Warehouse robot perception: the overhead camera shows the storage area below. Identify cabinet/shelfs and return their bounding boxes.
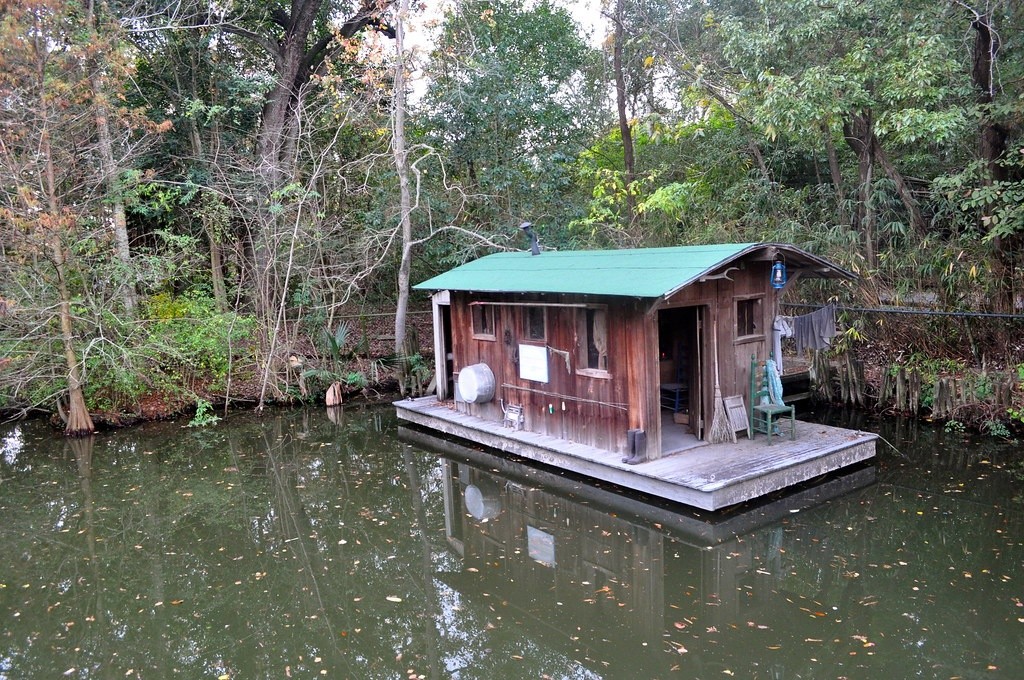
[659,381,687,414]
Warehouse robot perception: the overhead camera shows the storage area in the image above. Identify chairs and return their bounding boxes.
[747,353,794,446]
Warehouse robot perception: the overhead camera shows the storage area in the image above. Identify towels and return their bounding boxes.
[774,301,839,378]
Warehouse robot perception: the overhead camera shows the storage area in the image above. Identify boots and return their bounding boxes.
[622,429,646,464]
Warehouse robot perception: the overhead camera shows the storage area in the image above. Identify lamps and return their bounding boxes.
[770,249,787,289]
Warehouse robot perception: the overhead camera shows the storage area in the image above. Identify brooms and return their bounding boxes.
[706,320,738,445]
